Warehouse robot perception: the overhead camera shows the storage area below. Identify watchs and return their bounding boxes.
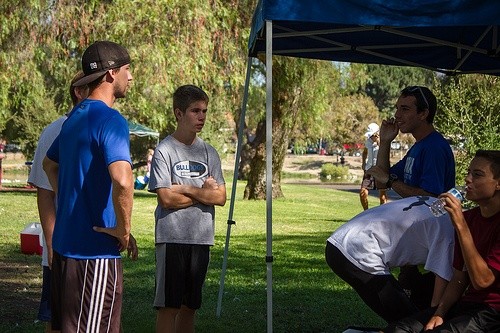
[386,173,398,189]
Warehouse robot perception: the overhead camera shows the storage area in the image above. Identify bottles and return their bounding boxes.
[429,186,470,217]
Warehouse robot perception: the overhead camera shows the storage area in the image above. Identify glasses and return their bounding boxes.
[401,86,429,109]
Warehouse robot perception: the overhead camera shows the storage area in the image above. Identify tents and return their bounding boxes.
[216,0,500,332]
[126,120,160,147]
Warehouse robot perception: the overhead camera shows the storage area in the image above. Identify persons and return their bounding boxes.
[423,148,500,333]
[149,84,227,333]
[362,86,456,333]
[389,140,411,158]
[28,70,94,332]
[42,40,134,332]
[325,194,457,333]
[146,148,155,179]
[360,122,387,213]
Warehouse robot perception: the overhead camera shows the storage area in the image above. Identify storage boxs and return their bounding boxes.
[20,222,44,255]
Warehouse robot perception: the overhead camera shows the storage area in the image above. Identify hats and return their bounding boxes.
[364,122,380,138]
[72,40,130,87]
